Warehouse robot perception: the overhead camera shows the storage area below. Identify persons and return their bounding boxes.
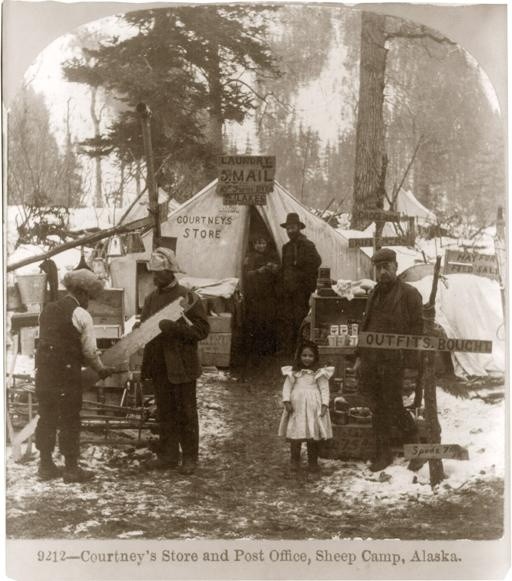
[278,340,336,472]
[33,268,115,484]
[270,212,322,361]
[230,232,282,378]
[133,247,210,475]
[345,248,429,471]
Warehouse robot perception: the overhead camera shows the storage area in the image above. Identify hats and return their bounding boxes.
[147,249,184,273]
[280,214,305,230]
[372,249,397,264]
[63,270,102,298]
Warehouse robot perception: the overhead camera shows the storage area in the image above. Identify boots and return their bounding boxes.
[404,431,429,471]
[368,431,395,472]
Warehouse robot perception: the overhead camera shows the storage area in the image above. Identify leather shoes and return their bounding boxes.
[180,460,196,475]
[145,459,180,470]
[38,464,63,482]
[62,470,97,484]
